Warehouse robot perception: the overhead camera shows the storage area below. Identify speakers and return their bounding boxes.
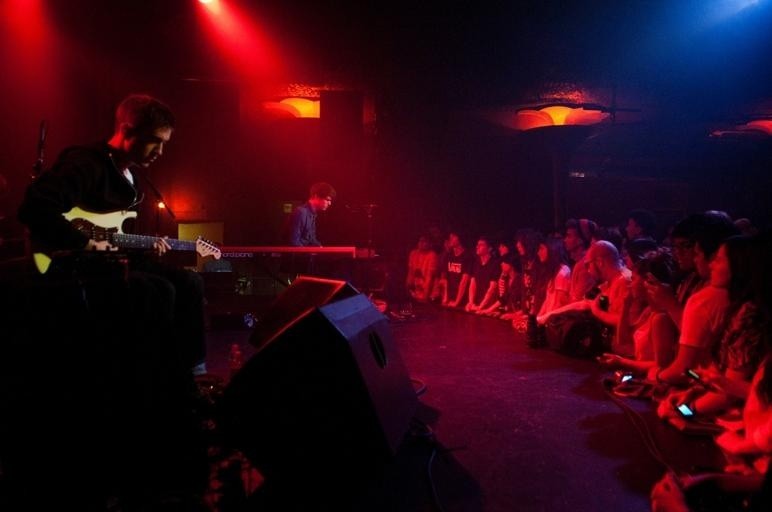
[554,318,600,357]
[219,293,421,511]
[248,272,360,354]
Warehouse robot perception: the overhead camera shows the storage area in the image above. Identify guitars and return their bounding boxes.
[61,206,221,276]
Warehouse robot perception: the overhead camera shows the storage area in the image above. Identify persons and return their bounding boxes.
[598,206,771,511]
[276,179,336,280]
[16,94,218,454]
[403,218,635,354]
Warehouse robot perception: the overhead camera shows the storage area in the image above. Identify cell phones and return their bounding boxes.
[687,368,702,382]
[620,373,634,382]
[673,404,695,418]
[638,267,653,285]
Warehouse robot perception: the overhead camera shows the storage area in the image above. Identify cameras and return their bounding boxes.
[585,287,608,308]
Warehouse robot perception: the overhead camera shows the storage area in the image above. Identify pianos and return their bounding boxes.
[215,241,360,263]
